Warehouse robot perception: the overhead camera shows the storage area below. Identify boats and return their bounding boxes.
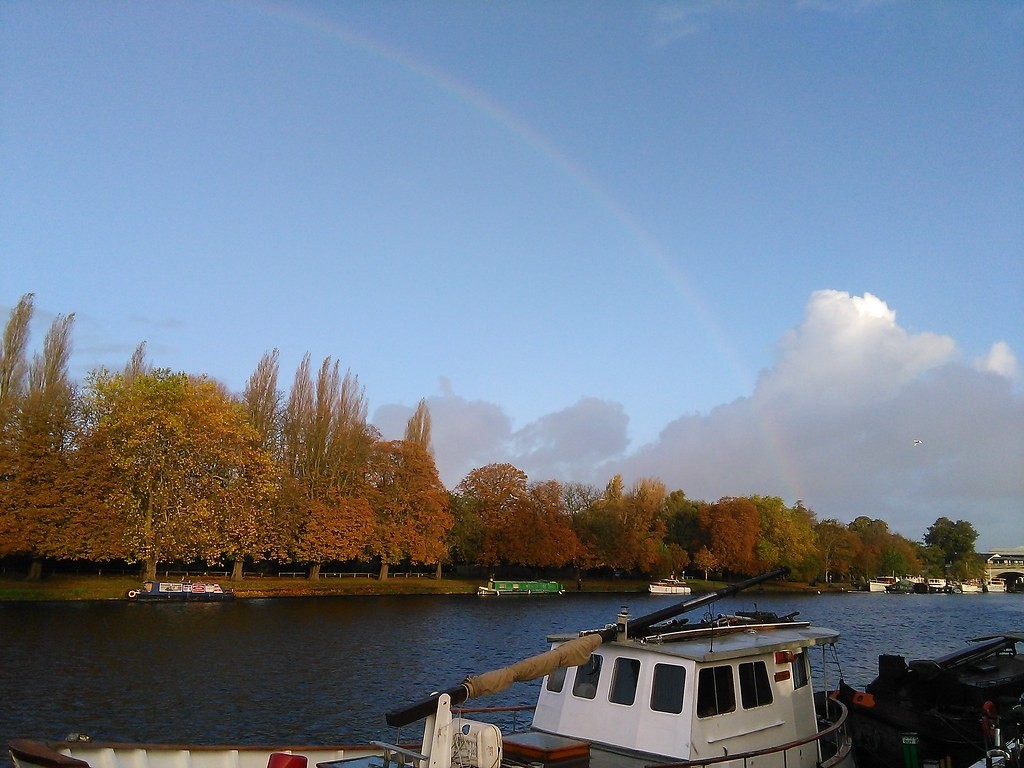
[648,571,691,594]
[478,574,565,595]
[869,565,1015,594]
[126,580,236,603]
[5,616,1023,768]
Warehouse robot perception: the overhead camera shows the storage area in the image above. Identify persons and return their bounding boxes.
[1010,692,1024,732]
[977,700,1007,748]
